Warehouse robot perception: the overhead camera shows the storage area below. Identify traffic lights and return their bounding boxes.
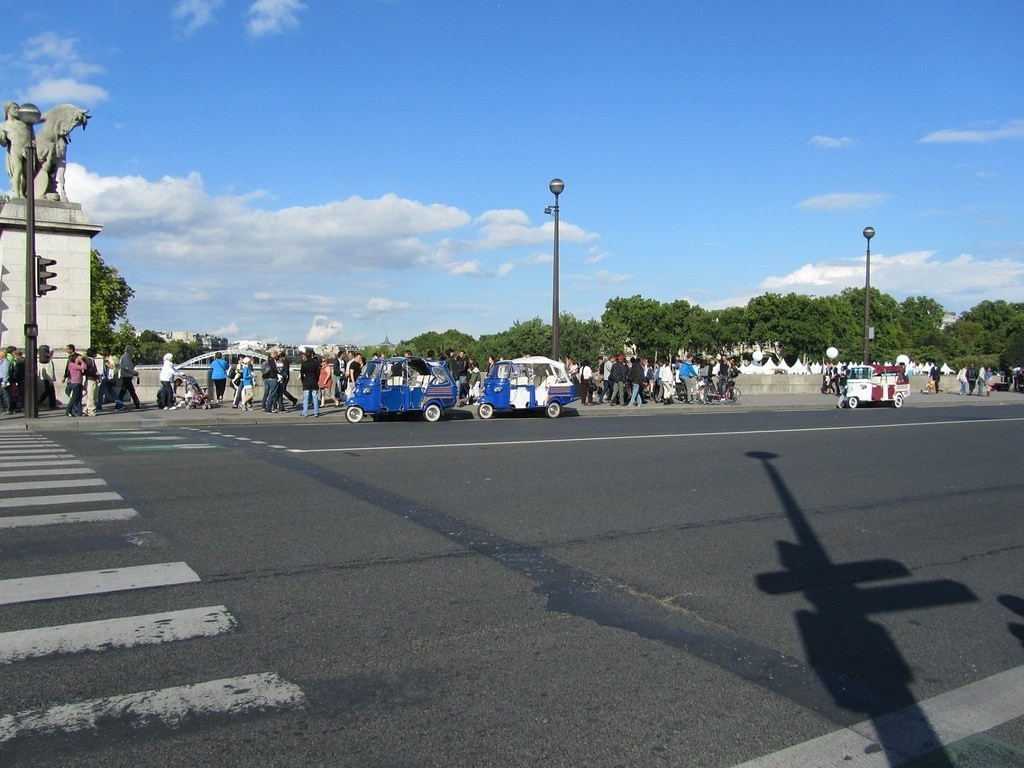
[38,257,56,296]
[39,345,51,363]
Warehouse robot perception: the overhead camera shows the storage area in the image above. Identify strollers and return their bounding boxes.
[182,375,212,410]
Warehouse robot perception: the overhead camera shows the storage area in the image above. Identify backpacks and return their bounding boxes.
[729,359,738,377]
[701,364,709,377]
[261,358,272,375]
[626,367,635,382]
[608,363,622,382]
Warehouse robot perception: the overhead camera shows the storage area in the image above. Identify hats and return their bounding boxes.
[243,357,251,363]
[445,349,454,356]
[618,355,624,360]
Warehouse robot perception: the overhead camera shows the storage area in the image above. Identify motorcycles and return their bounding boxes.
[846,365,911,409]
[346,355,459,423]
[477,356,574,419]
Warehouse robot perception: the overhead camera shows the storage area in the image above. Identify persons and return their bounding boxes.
[499,357,509,374]
[486,356,496,377]
[0,345,439,416]
[1005,366,1024,391]
[580,355,738,408]
[930,362,940,393]
[824,361,850,394]
[439,349,481,407]
[564,357,579,385]
[0,101,37,199]
[984,366,991,397]
[965,363,979,396]
[959,364,968,396]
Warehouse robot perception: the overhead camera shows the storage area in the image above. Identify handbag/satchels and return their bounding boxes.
[587,379,597,391]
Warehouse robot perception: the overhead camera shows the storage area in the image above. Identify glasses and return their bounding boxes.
[322,361,326,362]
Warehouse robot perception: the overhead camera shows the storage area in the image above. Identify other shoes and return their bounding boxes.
[629,403,633,407]
[164,407,168,409]
[664,399,669,405]
[293,399,298,407]
[599,400,604,403]
[319,405,325,408]
[96,406,103,411]
[65,412,73,416]
[335,400,340,407]
[217,400,219,403]
[219,396,222,401]
[279,409,289,414]
[2,410,13,415]
[959,393,961,394]
[232,404,238,408]
[667,398,674,404]
[689,398,695,403]
[135,405,147,410]
[473,401,480,406]
[986,391,991,397]
[50,406,59,409]
[262,407,265,411]
[610,400,613,405]
[239,404,246,410]
[117,405,126,411]
[169,406,177,410]
[463,400,469,405]
[638,404,646,407]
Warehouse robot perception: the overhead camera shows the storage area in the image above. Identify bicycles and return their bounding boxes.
[672,374,706,405]
[698,376,741,406]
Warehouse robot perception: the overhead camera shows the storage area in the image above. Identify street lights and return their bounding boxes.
[863,227,876,365]
[545,179,564,360]
[18,103,43,419]
